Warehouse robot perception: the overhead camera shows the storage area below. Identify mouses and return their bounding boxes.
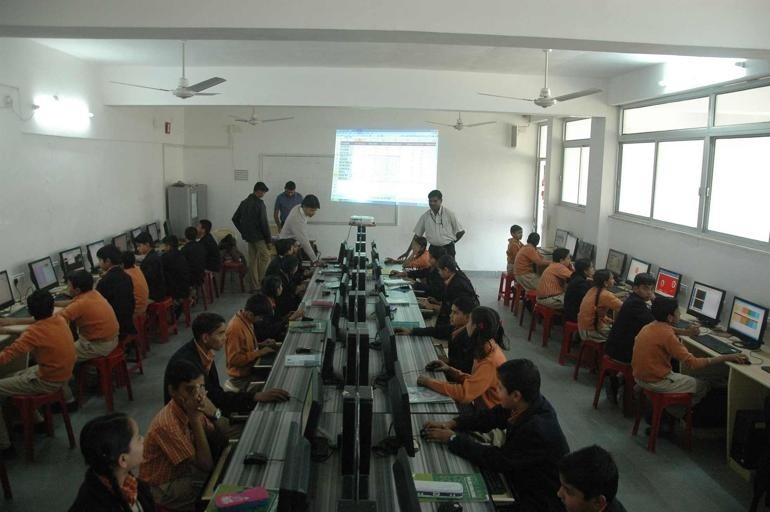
[295,344,312,353]
[243,451,268,465]
[322,290,330,296]
[399,284,410,289]
[742,358,751,365]
[437,501,463,512]
[301,315,315,322]
[420,426,430,438]
[425,363,440,370]
[319,263,329,268]
[315,278,325,283]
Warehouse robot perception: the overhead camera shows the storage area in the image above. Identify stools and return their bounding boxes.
[6,388,78,462]
[75,346,135,414]
[172,289,193,329]
[559,321,593,369]
[519,291,537,330]
[631,387,694,455]
[115,333,143,389]
[593,355,634,418]
[134,314,150,358]
[209,271,219,303]
[528,304,554,347]
[220,262,245,293]
[148,296,178,344]
[511,282,524,315]
[197,274,211,311]
[574,340,605,380]
[497,271,515,305]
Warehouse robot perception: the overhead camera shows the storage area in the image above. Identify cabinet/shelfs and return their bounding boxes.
[168,184,208,238]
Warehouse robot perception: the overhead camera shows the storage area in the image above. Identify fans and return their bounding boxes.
[423,111,497,131]
[233,107,294,125]
[110,39,225,99]
[478,47,603,108]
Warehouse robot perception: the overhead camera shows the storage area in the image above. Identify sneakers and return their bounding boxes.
[604,375,619,405]
[51,400,79,413]
[15,419,46,433]
[0,443,16,460]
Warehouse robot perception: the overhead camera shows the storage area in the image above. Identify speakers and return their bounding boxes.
[510,123,519,149]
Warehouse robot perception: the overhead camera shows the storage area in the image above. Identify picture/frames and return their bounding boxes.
[165,122,171,133]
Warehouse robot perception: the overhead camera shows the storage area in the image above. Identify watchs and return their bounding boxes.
[448,434,457,442]
[206,408,222,421]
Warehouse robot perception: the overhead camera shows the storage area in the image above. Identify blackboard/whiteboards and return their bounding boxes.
[258,154,398,227]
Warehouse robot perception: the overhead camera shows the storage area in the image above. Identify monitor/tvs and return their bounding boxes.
[727,296,769,349]
[146,222,160,243]
[654,267,682,299]
[337,240,348,268]
[299,366,329,457]
[371,257,382,281]
[339,272,349,300]
[130,226,142,241]
[370,246,379,263]
[86,239,105,274]
[370,240,376,249]
[163,219,172,237]
[564,234,578,258]
[59,246,85,277]
[374,274,389,297]
[320,320,337,377]
[686,280,727,326]
[112,233,128,253]
[605,248,628,276]
[574,239,595,262]
[391,445,422,512]
[276,421,319,512]
[28,255,59,291]
[329,290,344,329]
[375,291,391,328]
[378,315,398,376]
[340,257,349,279]
[0,270,15,311]
[625,257,651,289]
[383,360,420,458]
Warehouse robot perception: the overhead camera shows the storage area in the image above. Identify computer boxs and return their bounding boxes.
[348,290,356,321]
[690,386,728,431]
[730,408,766,471]
[357,290,366,322]
[355,242,366,252]
[358,385,374,475]
[352,269,358,290]
[353,253,366,269]
[345,321,357,385]
[358,270,366,291]
[358,322,370,386]
[341,386,357,475]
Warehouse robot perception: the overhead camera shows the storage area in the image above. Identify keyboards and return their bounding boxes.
[689,334,741,354]
[481,469,508,495]
[258,344,280,365]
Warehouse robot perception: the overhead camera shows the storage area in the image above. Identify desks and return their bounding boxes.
[252,301,460,413]
[222,411,496,512]
[673,307,770,482]
[301,258,418,304]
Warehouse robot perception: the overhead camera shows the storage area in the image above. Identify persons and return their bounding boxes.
[507,225,524,274]
[422,359,570,512]
[232,182,274,294]
[578,269,623,343]
[95,244,137,340]
[536,248,576,309]
[197,219,220,272]
[254,275,305,342]
[393,295,480,367]
[630,297,749,420]
[0,289,76,459]
[164,312,290,407]
[67,413,155,512]
[0,269,119,412]
[418,255,475,311]
[138,358,231,512]
[397,190,465,261]
[120,251,149,314]
[136,231,167,304]
[563,258,594,320]
[605,273,700,405]
[279,195,324,267]
[418,307,508,410]
[274,181,304,232]
[513,232,549,290]
[276,256,305,309]
[557,443,627,512]
[225,295,277,391]
[389,246,446,294]
[161,235,193,299]
[265,239,293,275]
[290,238,299,256]
[181,227,204,286]
[384,237,430,267]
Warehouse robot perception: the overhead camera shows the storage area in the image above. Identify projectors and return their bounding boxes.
[349,215,374,225]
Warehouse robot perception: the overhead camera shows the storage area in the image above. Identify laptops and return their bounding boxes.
[540,228,569,255]
